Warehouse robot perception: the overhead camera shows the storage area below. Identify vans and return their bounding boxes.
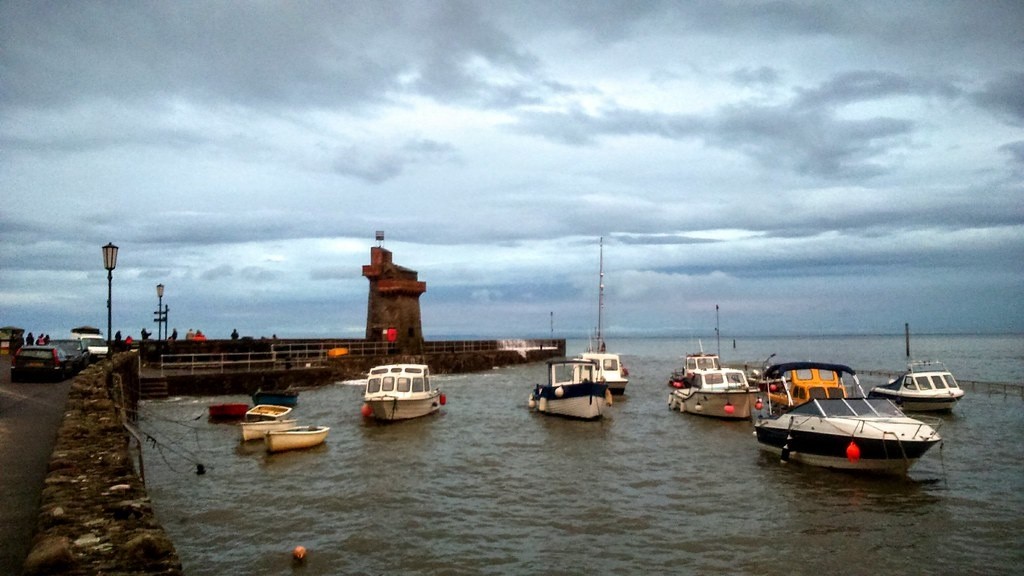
[69,326,111,358]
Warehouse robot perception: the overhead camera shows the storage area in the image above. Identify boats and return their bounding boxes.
[761,366,851,412]
[267,424,331,452]
[241,418,298,443]
[679,337,727,386]
[244,401,295,424]
[666,367,759,419]
[742,365,790,394]
[253,384,300,406]
[528,356,613,421]
[867,356,967,415]
[361,363,446,421]
[567,235,630,395]
[206,402,249,419]
[750,360,948,481]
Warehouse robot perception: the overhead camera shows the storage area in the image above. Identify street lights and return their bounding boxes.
[156,283,165,352]
[101,240,120,361]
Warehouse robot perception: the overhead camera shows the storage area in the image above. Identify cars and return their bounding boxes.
[9,344,77,382]
[48,338,90,370]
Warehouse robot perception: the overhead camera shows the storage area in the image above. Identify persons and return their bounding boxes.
[10,333,16,351]
[15,332,24,349]
[26,333,34,345]
[141,328,151,339]
[125,336,132,345]
[186,329,194,338]
[115,331,121,341]
[38,334,45,345]
[172,328,177,339]
[196,330,205,338]
[44,335,50,344]
[231,329,238,340]
